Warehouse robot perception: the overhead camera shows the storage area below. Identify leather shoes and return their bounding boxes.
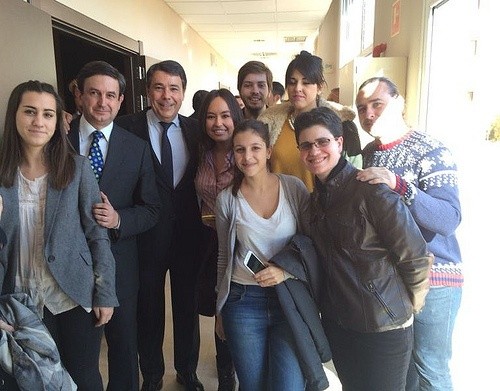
[141,379,163,391]
[176,374,204,391]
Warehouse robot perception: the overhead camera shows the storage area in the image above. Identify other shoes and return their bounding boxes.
[218,372,236,391]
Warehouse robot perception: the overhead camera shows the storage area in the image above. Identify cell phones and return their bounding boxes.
[243,249,268,274]
[201,214,216,222]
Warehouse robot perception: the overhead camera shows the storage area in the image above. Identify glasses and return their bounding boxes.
[297,137,338,151]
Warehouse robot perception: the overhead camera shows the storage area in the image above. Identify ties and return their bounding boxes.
[87,131,105,185]
[158,121,174,190]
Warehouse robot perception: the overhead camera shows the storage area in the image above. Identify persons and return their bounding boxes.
[0,49,464,391]
